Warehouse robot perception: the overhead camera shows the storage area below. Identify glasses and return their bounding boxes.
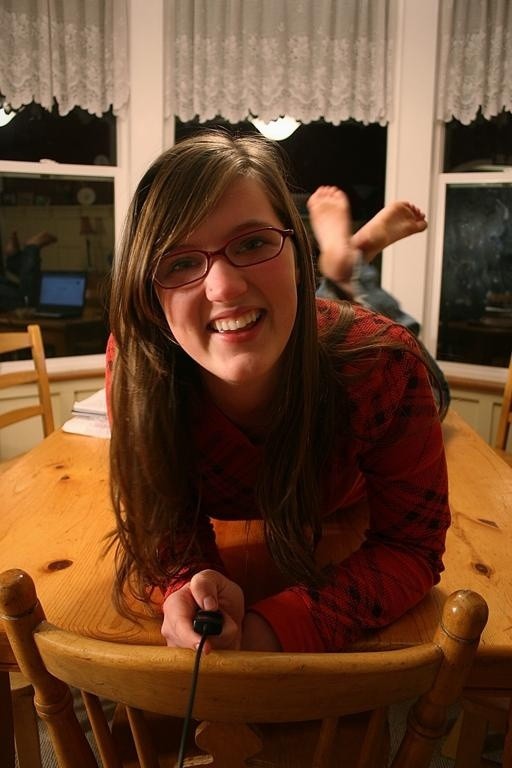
[148,226,295,289]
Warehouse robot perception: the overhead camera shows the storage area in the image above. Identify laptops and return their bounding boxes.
[32,270,88,318]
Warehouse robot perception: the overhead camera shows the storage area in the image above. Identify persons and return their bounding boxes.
[99,127,454,656]
[1,228,60,315]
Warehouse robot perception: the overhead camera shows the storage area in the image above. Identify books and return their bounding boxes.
[61,386,113,437]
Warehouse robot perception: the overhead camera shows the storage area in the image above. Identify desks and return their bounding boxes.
[1,363,512,738]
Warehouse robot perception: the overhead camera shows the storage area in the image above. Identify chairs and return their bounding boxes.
[492,361,512,463]
[1,563,492,767]
[2,322,55,461]
